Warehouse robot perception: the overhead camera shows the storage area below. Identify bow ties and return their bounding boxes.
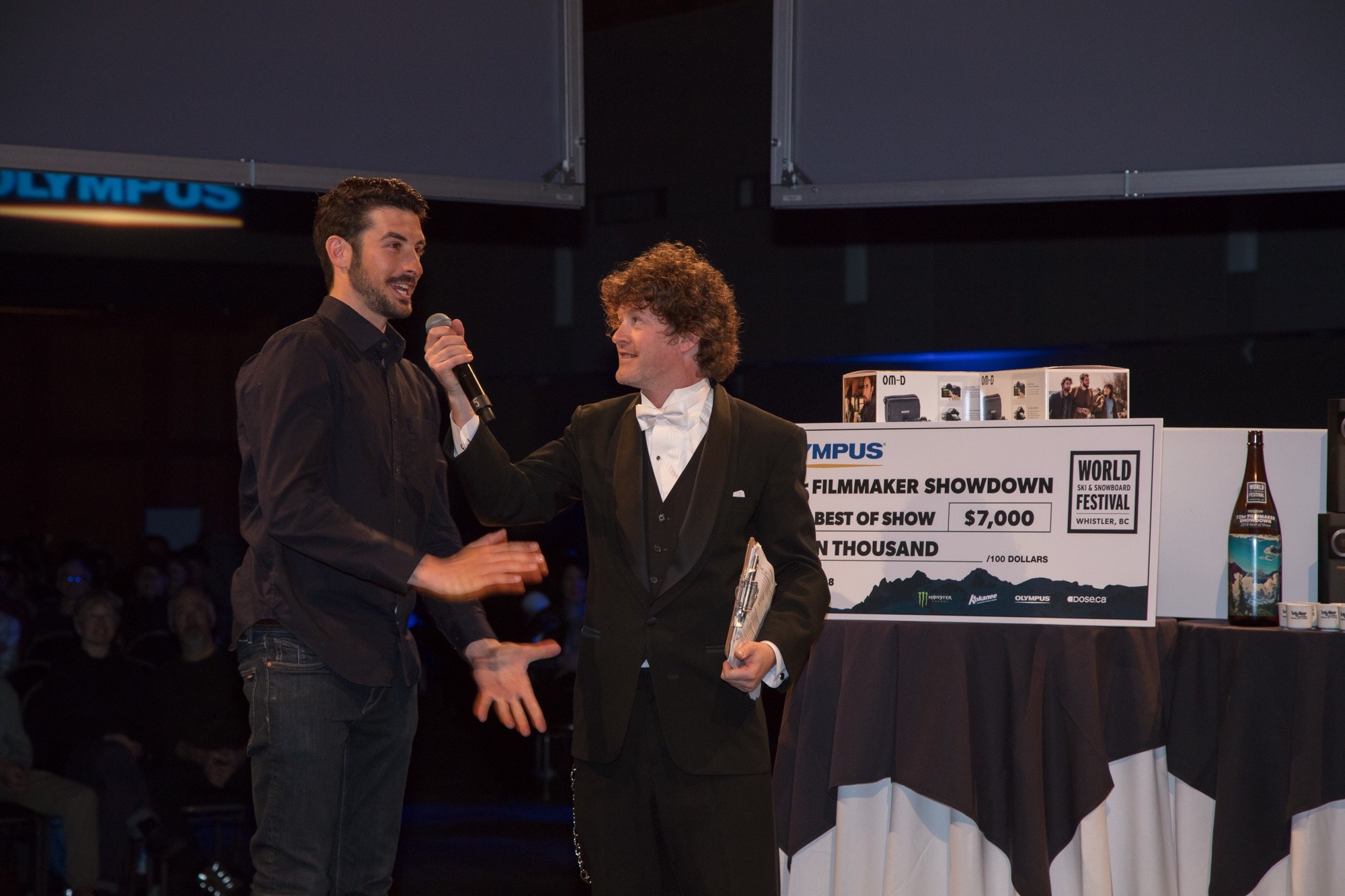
[636,401,688,431]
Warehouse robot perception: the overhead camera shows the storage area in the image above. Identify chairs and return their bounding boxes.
[0,803,247,896]
[530,663,574,801]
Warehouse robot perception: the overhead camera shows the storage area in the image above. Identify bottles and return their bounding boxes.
[1228,431,1282,627]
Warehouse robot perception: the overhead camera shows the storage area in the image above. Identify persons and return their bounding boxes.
[845,375,876,423]
[231,175,562,896]
[0,529,221,896]
[423,241,831,896]
[1049,374,1118,419]
[40,590,182,896]
[151,585,256,896]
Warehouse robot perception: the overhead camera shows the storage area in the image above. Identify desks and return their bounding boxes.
[770,614,1345,896]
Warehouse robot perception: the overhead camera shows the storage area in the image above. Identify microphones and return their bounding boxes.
[425,313,497,426]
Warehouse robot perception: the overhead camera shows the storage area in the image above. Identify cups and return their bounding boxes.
[1277,603,1293,627]
[1285,604,1313,628]
[1298,602,1320,627]
[1316,604,1339,631]
[1330,603,1345,605]
[1337,605,1345,633]
